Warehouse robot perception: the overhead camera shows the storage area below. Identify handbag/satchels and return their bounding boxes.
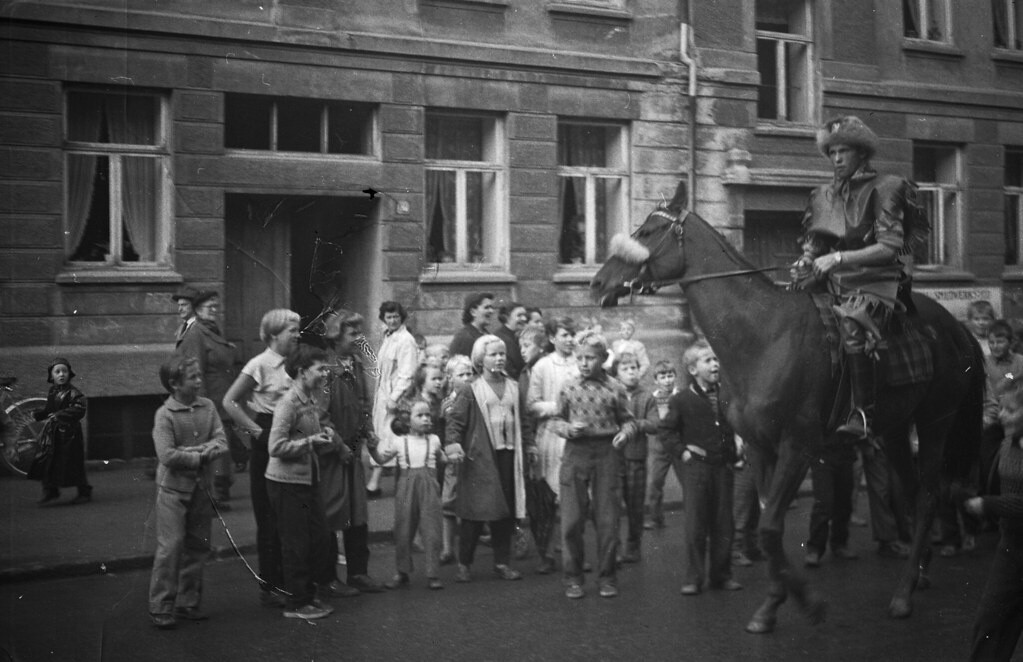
[26,420,48,479]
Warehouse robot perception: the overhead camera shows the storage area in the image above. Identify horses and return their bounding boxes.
[590,179,986,635]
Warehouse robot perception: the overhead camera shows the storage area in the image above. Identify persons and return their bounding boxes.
[32,357,97,509]
[149,353,229,630]
[546,330,637,598]
[365,300,420,500]
[785,115,908,442]
[174,287,1023,662]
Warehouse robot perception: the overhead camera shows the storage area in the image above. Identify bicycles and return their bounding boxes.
[0,376,49,477]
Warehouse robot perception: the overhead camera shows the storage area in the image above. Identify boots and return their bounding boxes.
[837,353,876,443]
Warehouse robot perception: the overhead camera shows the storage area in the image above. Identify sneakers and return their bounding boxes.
[283,596,335,619]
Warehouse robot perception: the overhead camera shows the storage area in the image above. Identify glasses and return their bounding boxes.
[199,304,219,308]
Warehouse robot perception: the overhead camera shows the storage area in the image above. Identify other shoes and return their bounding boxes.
[600,583,616,597]
[565,584,585,598]
[708,578,742,590]
[39,494,65,506]
[679,583,702,594]
[260,584,288,608]
[346,573,378,592]
[615,514,962,565]
[147,609,176,626]
[175,605,209,620]
[74,494,92,504]
[384,556,593,590]
[321,577,360,596]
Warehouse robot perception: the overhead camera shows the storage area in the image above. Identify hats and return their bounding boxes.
[172,286,199,301]
[817,115,880,164]
[192,291,213,309]
[47,358,76,383]
